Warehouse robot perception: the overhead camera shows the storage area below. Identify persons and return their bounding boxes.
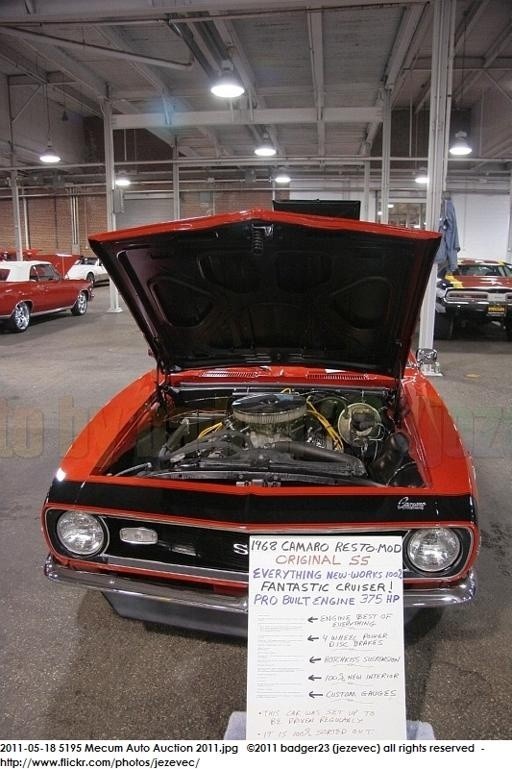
[23,252,28,261]
[1,251,11,261]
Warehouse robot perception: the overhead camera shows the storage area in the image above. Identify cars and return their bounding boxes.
[435,259,512,341]
[0,248,111,334]
[39,208,483,642]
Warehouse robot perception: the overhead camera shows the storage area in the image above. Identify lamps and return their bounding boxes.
[38,81,61,163]
[449,9,473,157]
[254,122,276,157]
[210,47,246,100]
